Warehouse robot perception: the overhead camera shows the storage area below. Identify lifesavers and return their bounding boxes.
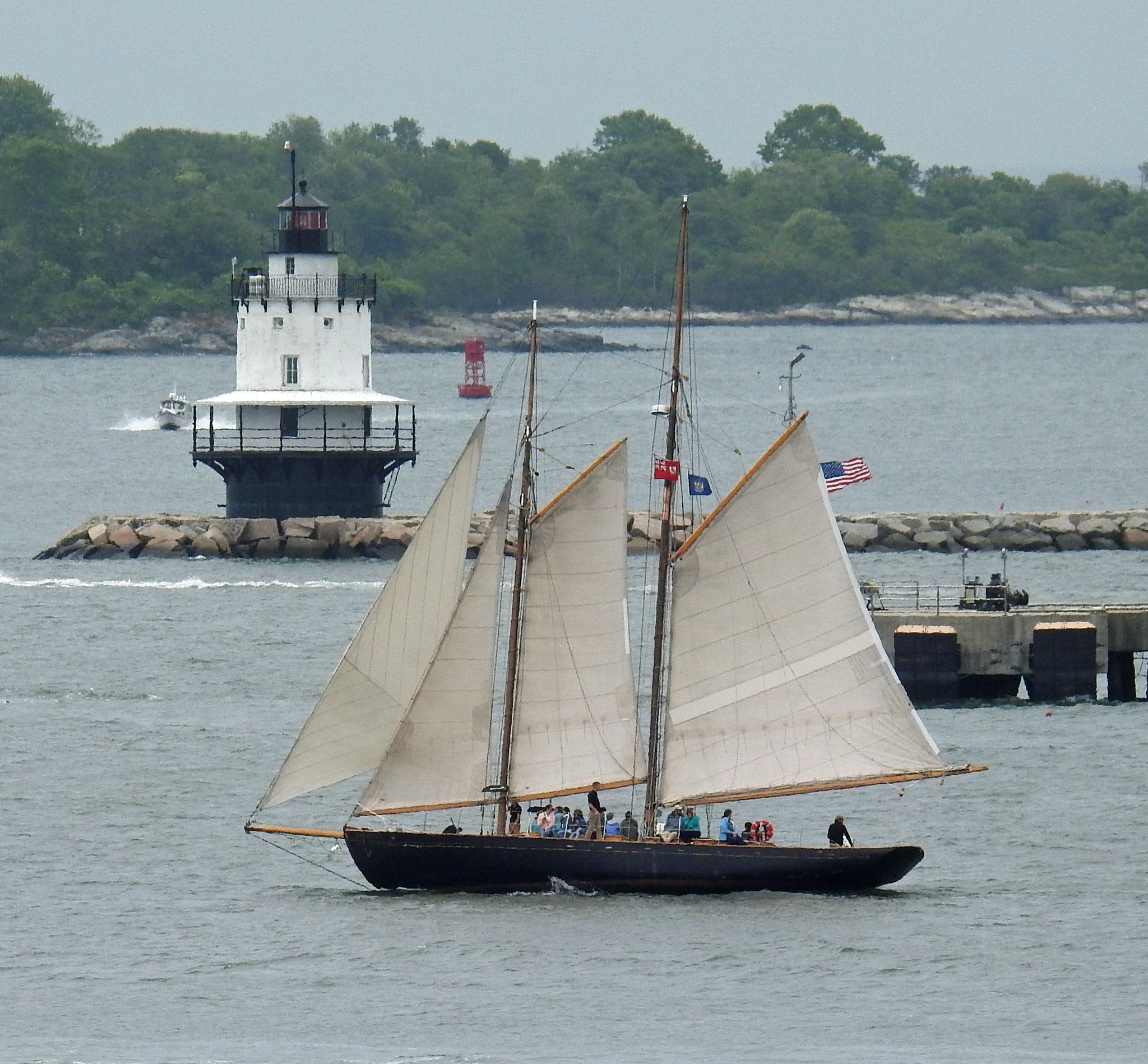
[750,819,773,843]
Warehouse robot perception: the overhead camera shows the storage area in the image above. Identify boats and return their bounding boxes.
[457,338,493,399]
[157,389,191,431]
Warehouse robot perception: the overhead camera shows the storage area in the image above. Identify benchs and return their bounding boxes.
[513,833,542,838]
[605,834,717,846]
[744,840,776,847]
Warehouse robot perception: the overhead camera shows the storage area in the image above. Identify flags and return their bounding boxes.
[688,474,712,496]
[654,458,680,481]
[820,457,872,492]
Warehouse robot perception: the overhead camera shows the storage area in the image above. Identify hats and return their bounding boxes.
[673,805,683,811]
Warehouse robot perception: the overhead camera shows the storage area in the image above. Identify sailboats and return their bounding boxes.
[244,192,991,894]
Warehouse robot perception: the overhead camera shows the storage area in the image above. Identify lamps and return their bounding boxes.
[232,256,237,279]
[651,405,669,415]
[284,141,293,153]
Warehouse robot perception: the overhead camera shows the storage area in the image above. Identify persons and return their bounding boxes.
[531,804,587,840]
[618,811,639,840]
[506,801,522,833]
[719,809,744,846]
[989,573,1004,586]
[827,815,853,848]
[658,804,686,842]
[583,781,603,841]
[680,807,701,843]
[606,812,620,836]
[741,822,753,839]
